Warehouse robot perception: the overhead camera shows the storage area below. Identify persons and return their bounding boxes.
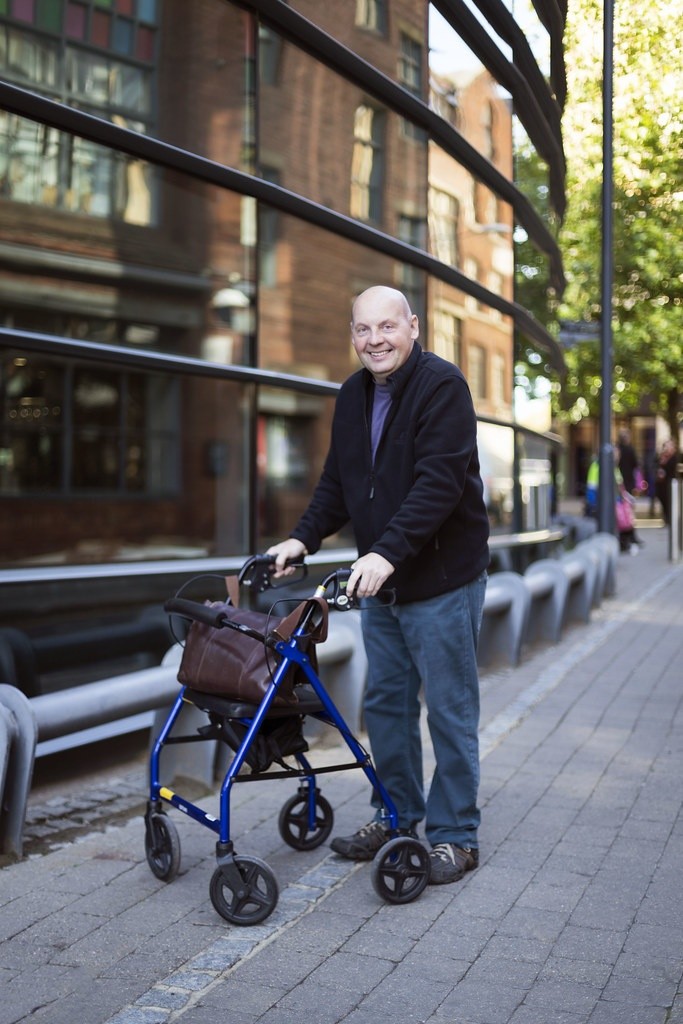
[267,284,491,886]
[584,427,683,557]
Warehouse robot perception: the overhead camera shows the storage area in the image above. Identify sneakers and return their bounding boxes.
[330,809,418,861]
[427,842,479,884]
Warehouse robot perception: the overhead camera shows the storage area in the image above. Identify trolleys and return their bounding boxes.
[141,553,434,928]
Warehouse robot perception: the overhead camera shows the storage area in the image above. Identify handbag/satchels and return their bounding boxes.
[177,575,329,708]
[615,501,635,533]
